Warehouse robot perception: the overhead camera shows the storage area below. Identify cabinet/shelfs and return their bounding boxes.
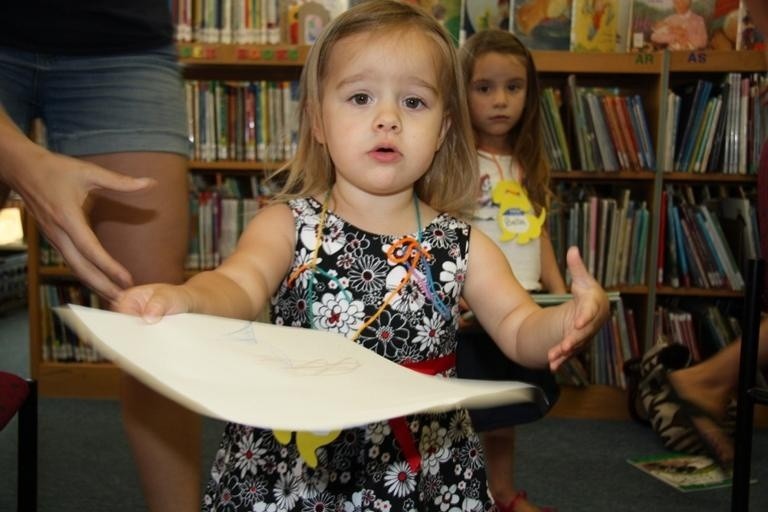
[27,1,766,421]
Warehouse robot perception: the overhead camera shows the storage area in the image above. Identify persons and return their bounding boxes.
[112,1,613,509]
[0,1,203,512]
[625,312,768,461]
[0,99,157,305]
[459,29,569,510]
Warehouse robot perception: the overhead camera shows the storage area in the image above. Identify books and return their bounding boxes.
[538,87,654,389]
[656,72,767,366]
[300,0,767,53]
[175,0,302,270]
[40,237,111,365]
